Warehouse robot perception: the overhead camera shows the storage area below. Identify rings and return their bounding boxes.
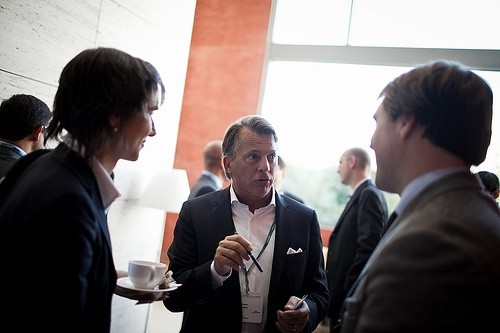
[294,324,297,332]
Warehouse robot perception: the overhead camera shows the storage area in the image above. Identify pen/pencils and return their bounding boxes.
[234,230,263,273]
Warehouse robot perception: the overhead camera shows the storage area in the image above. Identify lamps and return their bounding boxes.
[139,168,190,333]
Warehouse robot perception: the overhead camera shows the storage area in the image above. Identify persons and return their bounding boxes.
[186,140,225,202]
[325,147,388,333]
[275,156,305,205]
[0,47,166,333]
[0,94,52,193]
[166,113,330,333]
[341,60,500,333]
[477,171,500,201]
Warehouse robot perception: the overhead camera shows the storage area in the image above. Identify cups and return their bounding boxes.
[128,260,167,290]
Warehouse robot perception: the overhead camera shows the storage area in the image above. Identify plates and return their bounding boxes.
[117,276,178,293]
